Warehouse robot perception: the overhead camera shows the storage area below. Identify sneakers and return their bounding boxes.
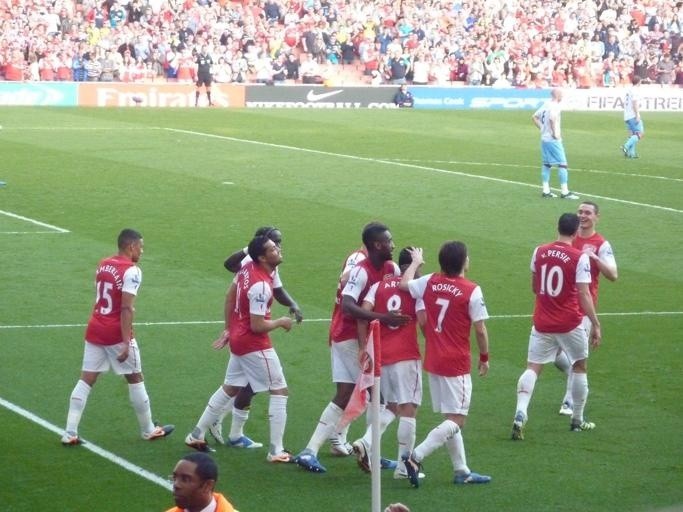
[559,401,574,416]
[562,192,580,202]
[184,430,426,489]
[512,415,524,441]
[142,424,176,441]
[571,422,597,432]
[543,192,558,199]
[454,469,493,485]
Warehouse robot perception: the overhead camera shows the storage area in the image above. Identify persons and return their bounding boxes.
[531,87,583,201]
[554,202,617,416]
[62,228,176,448]
[191,42,217,108]
[617,81,646,161]
[392,81,416,108]
[162,450,240,512]
[292,220,491,489]
[185,226,302,463]
[512,213,602,441]
[1,0,682,84]
